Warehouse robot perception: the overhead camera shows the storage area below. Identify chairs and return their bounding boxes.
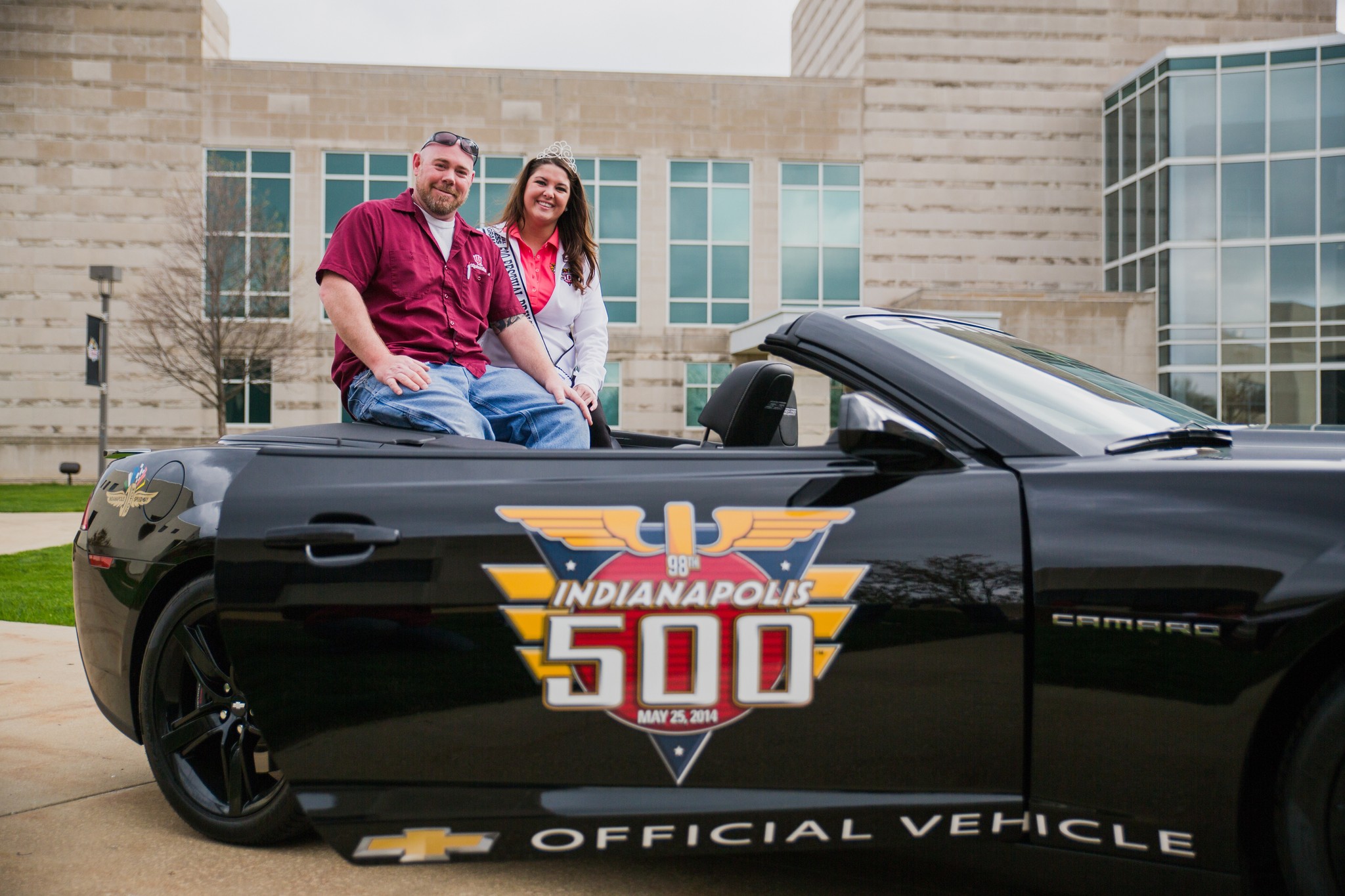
[672,360,799,453]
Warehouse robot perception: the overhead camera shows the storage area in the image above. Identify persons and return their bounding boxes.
[474,151,614,450]
[315,132,593,450]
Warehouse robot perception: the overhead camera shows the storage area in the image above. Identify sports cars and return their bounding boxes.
[68,305,1345,896]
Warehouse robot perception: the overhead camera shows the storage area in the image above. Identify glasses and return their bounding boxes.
[422,132,479,167]
